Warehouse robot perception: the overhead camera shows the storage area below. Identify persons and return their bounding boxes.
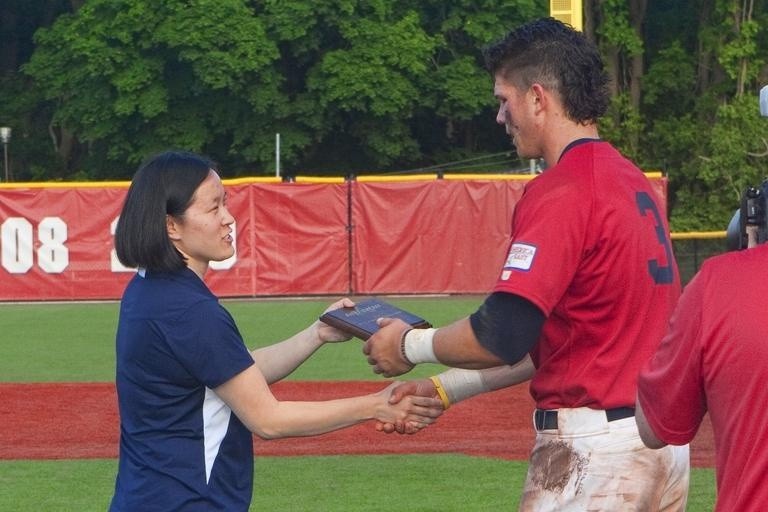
[106,151,448,512]
[363,18,691,512]
[633,239,768,512]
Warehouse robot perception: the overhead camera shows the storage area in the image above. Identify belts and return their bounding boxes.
[529,402,637,433]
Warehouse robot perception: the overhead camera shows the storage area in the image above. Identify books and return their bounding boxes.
[318,297,432,343]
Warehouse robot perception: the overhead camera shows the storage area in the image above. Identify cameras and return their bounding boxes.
[741,186,766,227]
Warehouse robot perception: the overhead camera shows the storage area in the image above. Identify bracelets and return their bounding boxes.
[430,376,450,409]
[401,325,439,369]
